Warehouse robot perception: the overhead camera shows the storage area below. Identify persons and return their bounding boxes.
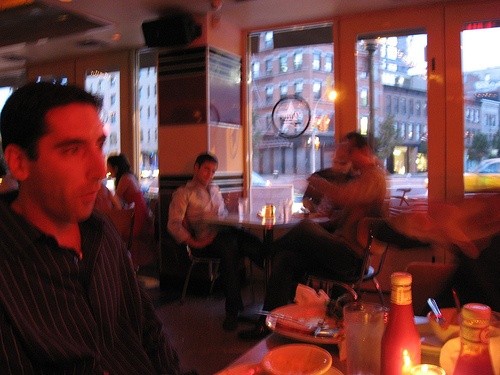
[237,131,391,343]
[0,80,181,375]
[167,154,264,331]
[106,154,154,272]
[93,181,131,237]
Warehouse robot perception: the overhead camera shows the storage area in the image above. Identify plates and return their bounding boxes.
[438,337,500,375]
[417,334,442,356]
[214,362,345,375]
[266,301,343,344]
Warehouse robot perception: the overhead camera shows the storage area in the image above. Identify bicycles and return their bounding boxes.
[391,187,411,208]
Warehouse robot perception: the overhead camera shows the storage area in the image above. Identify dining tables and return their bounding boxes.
[212,319,447,375]
[203,214,331,316]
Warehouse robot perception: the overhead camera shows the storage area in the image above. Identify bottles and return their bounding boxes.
[381,271,421,375]
[451,303,496,375]
[263,201,274,219]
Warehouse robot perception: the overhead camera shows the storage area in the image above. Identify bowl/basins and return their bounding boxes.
[264,344,332,375]
[427,307,500,344]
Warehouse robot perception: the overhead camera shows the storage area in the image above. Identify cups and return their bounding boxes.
[409,363,448,375]
[279,201,292,222]
[238,197,250,218]
[343,302,389,375]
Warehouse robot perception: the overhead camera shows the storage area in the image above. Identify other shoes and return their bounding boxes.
[223,317,238,331]
[237,321,268,342]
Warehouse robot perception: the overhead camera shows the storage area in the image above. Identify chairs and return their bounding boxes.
[179,214,222,305]
[302,216,389,308]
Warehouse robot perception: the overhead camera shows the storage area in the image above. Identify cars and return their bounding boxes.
[140,171,304,203]
[424,158,500,193]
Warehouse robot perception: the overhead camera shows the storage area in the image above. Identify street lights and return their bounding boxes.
[311,91,337,174]
[364,39,379,150]
